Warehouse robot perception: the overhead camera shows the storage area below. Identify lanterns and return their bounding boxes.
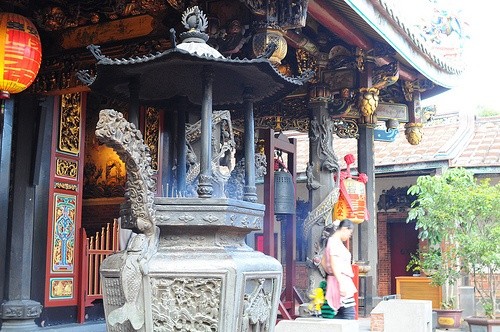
[331,178,369,225]
[0,11,44,99]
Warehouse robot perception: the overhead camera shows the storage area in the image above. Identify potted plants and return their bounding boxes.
[354,259,370,277]
[432,297,465,330]
[404,168,500,332]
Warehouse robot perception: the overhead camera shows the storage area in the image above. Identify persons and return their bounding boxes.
[314,220,360,319]
